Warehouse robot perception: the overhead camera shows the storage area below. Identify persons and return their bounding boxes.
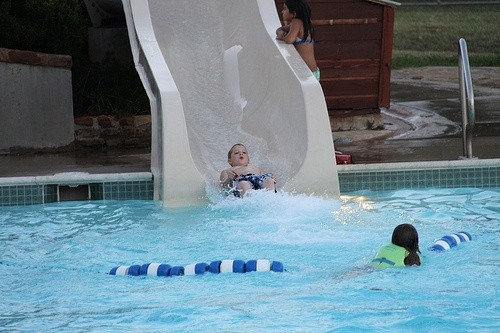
[276,0,320,82]
[219,143,277,200]
[339,223,421,280]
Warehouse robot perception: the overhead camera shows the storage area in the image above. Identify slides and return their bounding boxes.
[122,0,342,209]
